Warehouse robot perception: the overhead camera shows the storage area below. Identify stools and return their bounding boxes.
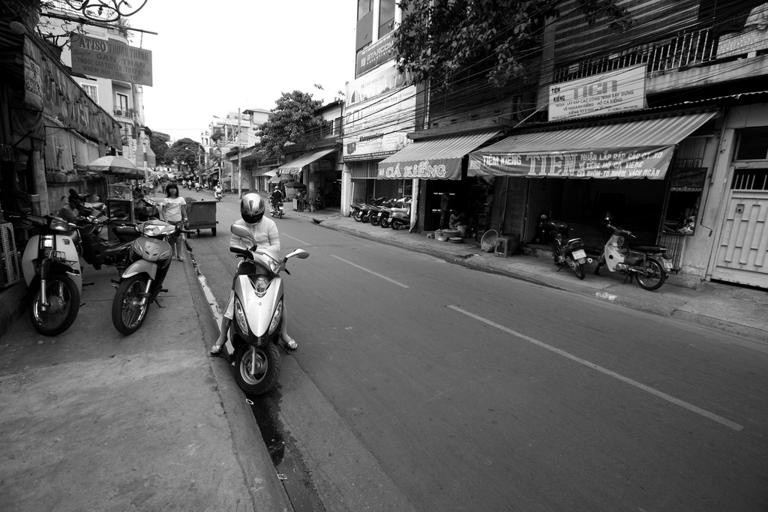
[495,236,513,257]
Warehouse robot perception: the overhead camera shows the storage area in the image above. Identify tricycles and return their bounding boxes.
[182,199,218,238]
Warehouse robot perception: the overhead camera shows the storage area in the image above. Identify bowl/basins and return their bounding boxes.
[479,228,498,252]
[450,237,462,243]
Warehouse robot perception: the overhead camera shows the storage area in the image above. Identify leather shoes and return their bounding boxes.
[281,334,298,350]
[211,337,228,354]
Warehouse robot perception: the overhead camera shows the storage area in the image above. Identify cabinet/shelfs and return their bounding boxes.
[109,183,132,200]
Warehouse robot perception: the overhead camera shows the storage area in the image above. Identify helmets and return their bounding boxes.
[240,193,265,224]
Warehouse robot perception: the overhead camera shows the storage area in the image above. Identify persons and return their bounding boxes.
[270,186,284,213]
[159,184,189,262]
[58,195,101,271]
[213,182,224,197]
[438,191,451,229]
[210,193,298,354]
[449,211,464,229]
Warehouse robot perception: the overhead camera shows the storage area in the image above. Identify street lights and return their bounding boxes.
[213,114,237,145]
[186,148,197,161]
[243,109,275,116]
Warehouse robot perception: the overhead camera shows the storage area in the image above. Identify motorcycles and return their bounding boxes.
[541,214,591,281]
[60,190,145,271]
[5,209,89,337]
[181,182,224,200]
[349,194,411,231]
[112,207,200,335]
[268,197,284,220]
[593,214,676,291]
[224,225,310,394]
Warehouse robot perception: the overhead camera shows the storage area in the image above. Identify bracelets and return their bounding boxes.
[184,218,187,220]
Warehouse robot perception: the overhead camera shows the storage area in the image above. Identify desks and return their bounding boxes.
[106,198,134,237]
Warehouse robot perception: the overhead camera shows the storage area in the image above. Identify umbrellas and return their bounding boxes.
[88,155,145,199]
